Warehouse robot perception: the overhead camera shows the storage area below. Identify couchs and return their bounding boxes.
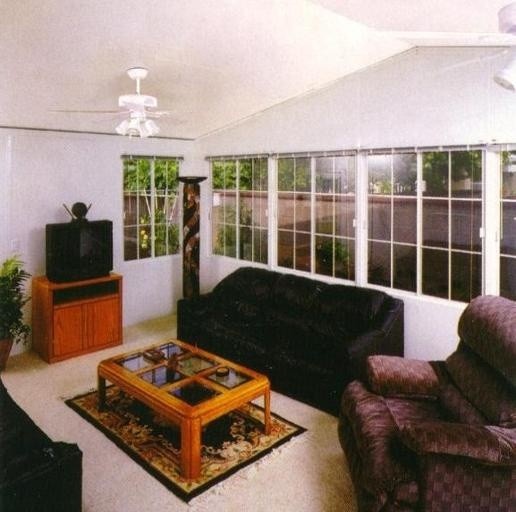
[0,375,83,512]
[176,266,405,418]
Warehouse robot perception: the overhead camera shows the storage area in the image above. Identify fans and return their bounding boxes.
[442,2,516,72]
[45,67,202,119]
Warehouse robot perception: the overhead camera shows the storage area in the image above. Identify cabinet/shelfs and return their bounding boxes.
[30,274,123,364]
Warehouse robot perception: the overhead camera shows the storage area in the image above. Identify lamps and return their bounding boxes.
[114,119,162,139]
[492,54,516,92]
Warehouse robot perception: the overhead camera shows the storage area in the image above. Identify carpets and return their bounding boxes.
[64,366,309,504]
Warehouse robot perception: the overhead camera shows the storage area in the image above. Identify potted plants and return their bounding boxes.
[0,253,33,372]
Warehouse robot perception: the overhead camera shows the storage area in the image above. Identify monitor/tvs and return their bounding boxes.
[46,221,112,283]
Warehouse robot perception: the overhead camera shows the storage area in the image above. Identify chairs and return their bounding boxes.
[336,293,516,512]
